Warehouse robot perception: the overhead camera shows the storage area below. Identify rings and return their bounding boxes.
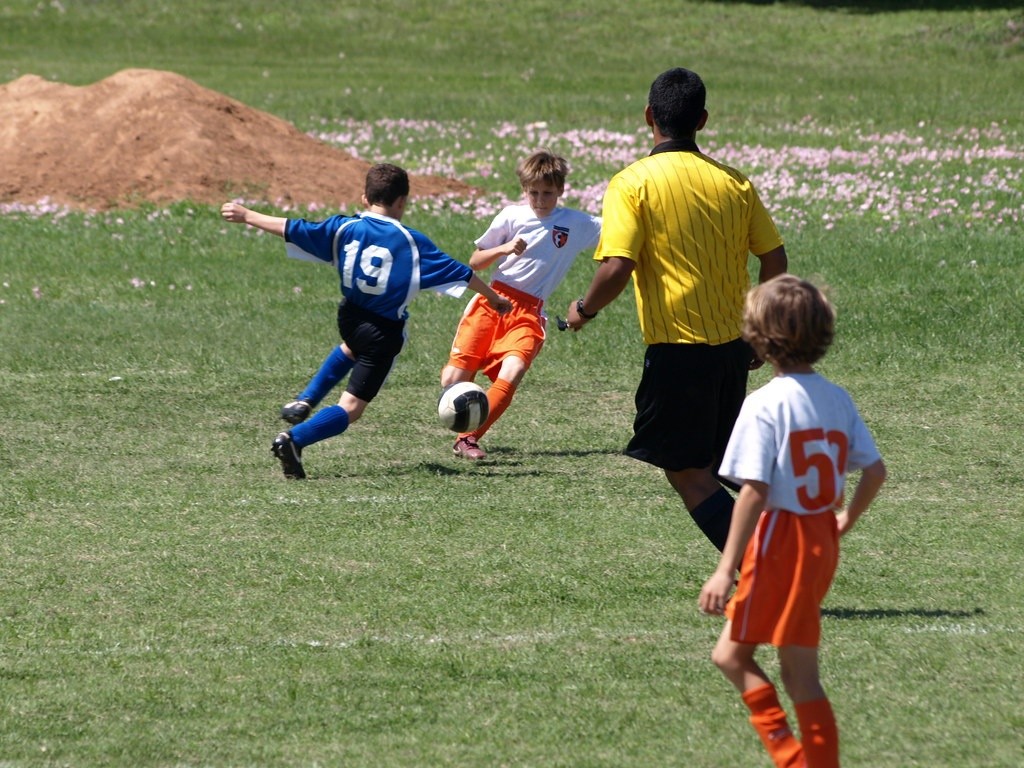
[567,324,570,328]
[566,319,568,323]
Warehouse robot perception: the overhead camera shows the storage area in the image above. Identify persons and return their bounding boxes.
[698,273,888,768]
[441,153,602,460]
[568,68,787,574]
[221,163,514,480]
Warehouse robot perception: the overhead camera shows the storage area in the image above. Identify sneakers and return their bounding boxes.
[452,435,487,459]
[281,401,312,425]
[271,431,306,480]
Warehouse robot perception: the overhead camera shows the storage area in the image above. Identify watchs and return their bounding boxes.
[576,299,597,319]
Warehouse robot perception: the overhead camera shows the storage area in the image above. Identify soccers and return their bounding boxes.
[436,381,490,434]
